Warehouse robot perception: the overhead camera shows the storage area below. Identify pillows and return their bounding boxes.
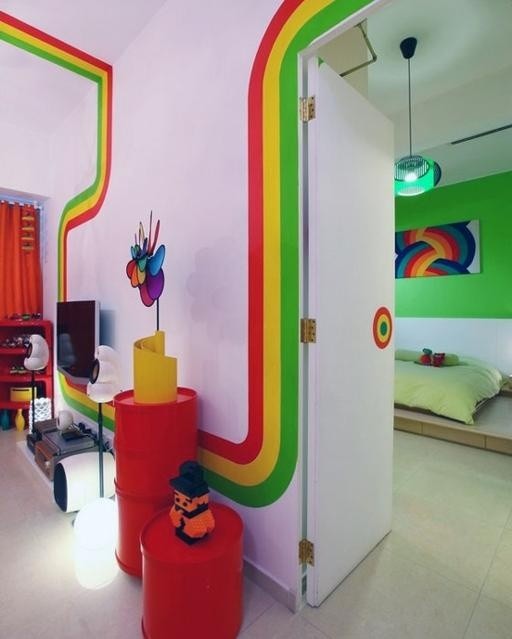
[414,352,459,366]
[395,349,422,360]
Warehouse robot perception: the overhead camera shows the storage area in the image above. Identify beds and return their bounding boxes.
[395,358,511,426]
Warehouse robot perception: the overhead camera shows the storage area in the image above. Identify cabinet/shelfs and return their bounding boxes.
[0,319,53,428]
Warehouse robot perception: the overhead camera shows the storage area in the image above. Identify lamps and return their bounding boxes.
[395,37,442,197]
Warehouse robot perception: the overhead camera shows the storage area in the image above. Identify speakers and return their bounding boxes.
[53,451,116,514]
[54,410,74,432]
[85,344,122,404]
[24,334,51,372]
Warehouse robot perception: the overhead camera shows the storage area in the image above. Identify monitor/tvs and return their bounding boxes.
[54,299,101,387]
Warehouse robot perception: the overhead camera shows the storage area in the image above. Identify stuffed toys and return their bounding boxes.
[420,347,433,364]
[433,351,447,368]
